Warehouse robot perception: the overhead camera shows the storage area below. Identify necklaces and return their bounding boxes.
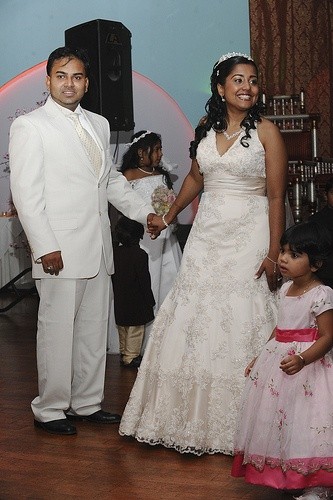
[138,168,155,174]
[289,280,315,296]
[222,129,243,139]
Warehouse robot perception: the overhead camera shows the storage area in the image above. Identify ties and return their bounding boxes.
[68,114,103,180]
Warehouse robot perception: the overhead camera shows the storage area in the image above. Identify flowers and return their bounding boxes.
[151,185,176,217]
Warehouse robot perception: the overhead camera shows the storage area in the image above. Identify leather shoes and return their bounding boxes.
[33,417,77,436]
[63,407,120,426]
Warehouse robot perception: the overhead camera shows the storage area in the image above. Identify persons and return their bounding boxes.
[118,52,295,456]
[8,47,157,435]
[231,220,333,500]
[107,131,183,357]
[110,216,157,368]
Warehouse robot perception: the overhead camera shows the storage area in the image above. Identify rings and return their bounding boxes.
[48,266,53,270]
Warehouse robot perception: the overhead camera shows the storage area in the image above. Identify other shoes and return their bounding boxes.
[123,355,143,368]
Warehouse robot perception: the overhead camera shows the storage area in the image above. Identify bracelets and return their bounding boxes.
[267,257,278,273]
[162,215,168,226]
[299,355,306,365]
